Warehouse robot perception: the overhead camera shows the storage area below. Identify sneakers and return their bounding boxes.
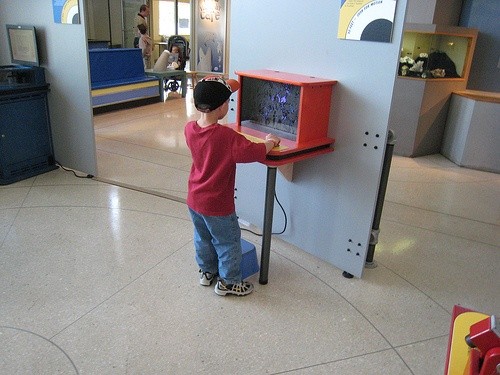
[199,269,217,286]
[215,279,254,296]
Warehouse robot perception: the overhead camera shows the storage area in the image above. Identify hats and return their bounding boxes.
[193,78,240,113]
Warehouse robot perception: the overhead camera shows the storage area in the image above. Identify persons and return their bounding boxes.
[184,74,280,297]
[134,5,150,48]
[172,45,182,70]
[138,24,152,69]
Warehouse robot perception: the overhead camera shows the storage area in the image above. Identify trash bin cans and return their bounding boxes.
[0,65,59,186]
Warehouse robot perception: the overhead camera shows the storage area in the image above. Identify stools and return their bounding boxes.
[186,71,199,90]
[238,237,260,281]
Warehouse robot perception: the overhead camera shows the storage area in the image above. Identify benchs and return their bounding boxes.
[88,47,160,111]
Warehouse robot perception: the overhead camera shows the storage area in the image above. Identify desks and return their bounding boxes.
[439,89,500,174]
[219,123,336,285]
[0,65,59,186]
[146,70,186,102]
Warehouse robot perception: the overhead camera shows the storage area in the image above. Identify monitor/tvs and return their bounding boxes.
[5,23,40,68]
